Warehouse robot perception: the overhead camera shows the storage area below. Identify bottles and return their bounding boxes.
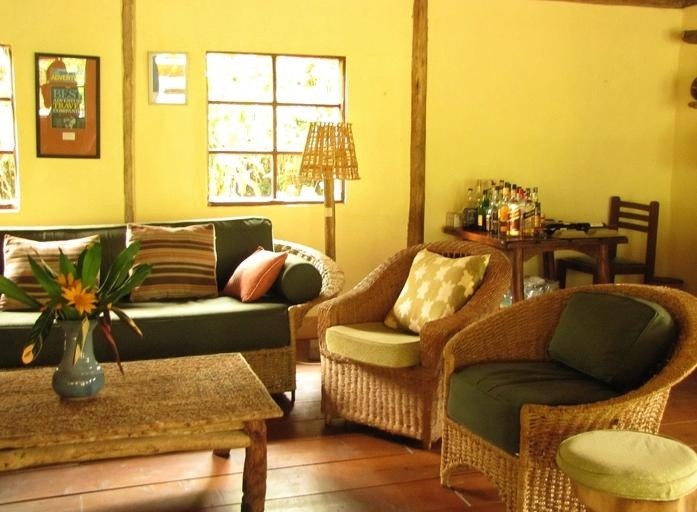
[462,178,542,238]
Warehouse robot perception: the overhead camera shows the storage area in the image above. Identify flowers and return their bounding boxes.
[0,242,153,380]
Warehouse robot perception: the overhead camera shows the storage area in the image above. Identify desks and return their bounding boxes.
[443,226,630,304]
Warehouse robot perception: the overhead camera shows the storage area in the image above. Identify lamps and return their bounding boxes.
[298,120,360,264]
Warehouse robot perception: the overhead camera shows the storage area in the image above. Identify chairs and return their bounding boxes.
[555,197,660,289]
[440,284,697,512]
[317,240,513,451]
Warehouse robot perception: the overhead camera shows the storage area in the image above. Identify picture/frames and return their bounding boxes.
[146,50,188,106]
[34,51,100,159]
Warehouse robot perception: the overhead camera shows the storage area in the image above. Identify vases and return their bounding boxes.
[52,318,106,401]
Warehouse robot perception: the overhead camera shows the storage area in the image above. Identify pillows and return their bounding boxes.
[0,234,101,310]
[125,222,218,302]
[546,291,678,392]
[264,253,323,303]
[383,247,491,335]
[224,246,288,303]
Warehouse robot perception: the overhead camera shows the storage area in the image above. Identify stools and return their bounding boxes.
[556,430,697,512]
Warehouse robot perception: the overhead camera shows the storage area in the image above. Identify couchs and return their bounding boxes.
[0,216,345,402]
